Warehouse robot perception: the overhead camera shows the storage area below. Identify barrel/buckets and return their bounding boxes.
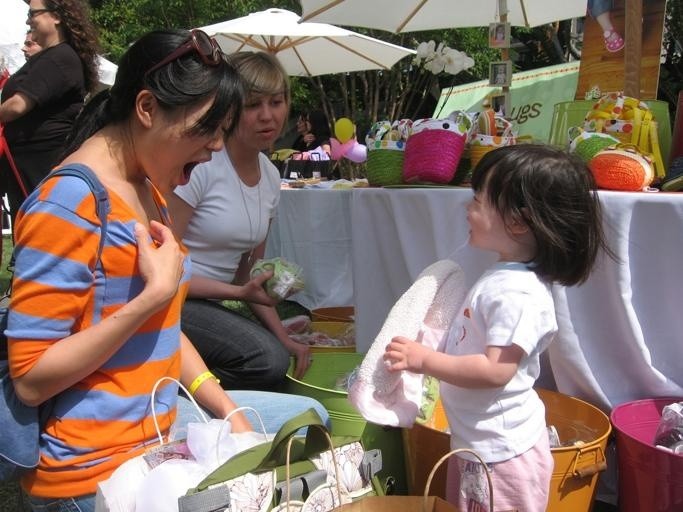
[282,342,410,447]
[412,385,612,511]
[610,396,678,512]
[314,305,356,320]
[283,317,361,350]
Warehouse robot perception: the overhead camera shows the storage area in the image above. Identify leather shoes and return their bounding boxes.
[478,111,496,136]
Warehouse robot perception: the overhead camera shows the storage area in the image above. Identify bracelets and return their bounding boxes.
[188,369,223,394]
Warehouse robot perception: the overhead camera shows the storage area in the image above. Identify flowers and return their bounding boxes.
[397,40,475,120]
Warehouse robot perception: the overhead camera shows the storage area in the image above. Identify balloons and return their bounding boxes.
[334,118,354,143]
[347,144,366,163]
[329,138,342,159]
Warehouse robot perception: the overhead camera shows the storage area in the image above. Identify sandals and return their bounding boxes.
[601,26,624,53]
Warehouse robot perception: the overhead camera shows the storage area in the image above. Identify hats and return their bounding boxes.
[347,260,468,429]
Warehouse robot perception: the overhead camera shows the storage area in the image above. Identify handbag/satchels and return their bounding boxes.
[365,118,412,186]
[403,110,473,185]
[286,424,493,511]
[97,377,277,512]
[269,152,285,178]
[285,152,309,179]
[586,142,654,192]
[305,152,328,177]
[470,117,532,171]
[568,126,621,164]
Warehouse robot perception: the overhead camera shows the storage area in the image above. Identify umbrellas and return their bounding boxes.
[185,9,418,78]
[296,0,590,124]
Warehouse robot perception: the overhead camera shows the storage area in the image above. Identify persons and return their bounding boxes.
[303,110,331,155]
[1,1,100,245]
[21,31,40,59]
[294,114,308,151]
[6,30,253,512]
[173,52,311,391]
[383,144,609,512]
[588,0,625,53]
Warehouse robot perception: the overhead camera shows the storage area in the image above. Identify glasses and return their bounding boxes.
[147,30,223,75]
[27,8,53,16]
